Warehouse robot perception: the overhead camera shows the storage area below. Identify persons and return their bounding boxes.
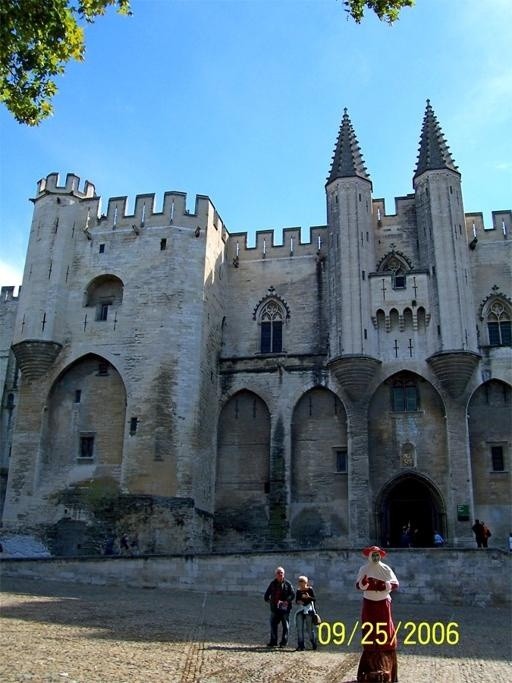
[357,544,400,670]
[296,575,317,649]
[263,567,295,646]
[398,516,511,552]
[104,532,139,555]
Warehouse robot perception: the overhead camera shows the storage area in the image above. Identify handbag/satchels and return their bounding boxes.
[313,614,321,625]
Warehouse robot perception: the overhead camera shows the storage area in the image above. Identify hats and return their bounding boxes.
[364,546,385,557]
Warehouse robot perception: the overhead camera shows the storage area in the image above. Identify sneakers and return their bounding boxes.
[280,644,287,648]
[267,643,277,646]
[296,647,305,650]
[313,639,317,650]
